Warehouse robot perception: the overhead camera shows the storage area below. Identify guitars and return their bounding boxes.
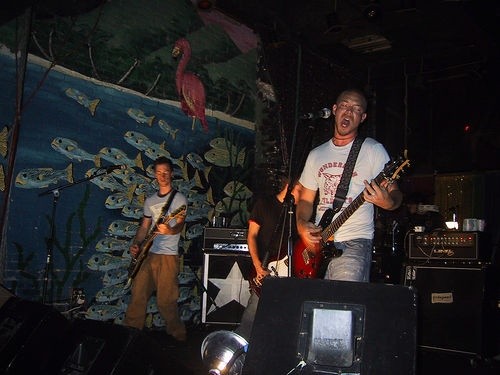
[124,201,189,291]
[245,253,290,299]
[293,149,416,278]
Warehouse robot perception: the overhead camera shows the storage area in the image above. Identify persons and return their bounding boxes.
[235,174,301,342]
[295,89,405,283]
[123,156,187,345]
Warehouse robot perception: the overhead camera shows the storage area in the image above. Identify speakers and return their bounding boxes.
[401,260,500,375]
[0,283,193,375]
[242,277,416,375]
[202,251,256,325]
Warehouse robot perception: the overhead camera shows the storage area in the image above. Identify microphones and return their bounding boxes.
[302,108,332,118]
[101,164,127,170]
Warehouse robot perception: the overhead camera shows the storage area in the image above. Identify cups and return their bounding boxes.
[463,219,484,232]
